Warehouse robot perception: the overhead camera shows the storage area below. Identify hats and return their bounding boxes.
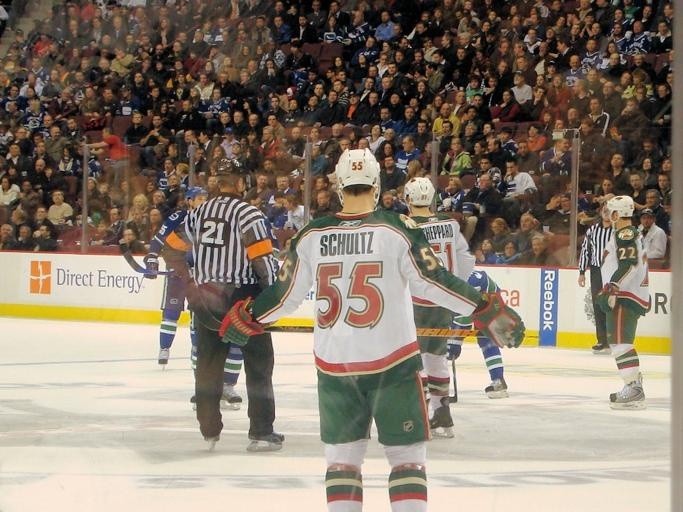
[640,208,656,218]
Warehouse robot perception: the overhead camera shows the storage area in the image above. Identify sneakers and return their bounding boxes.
[248,429,285,443]
[222,383,242,404]
[158,348,169,364]
[592,342,609,350]
[485,377,507,392]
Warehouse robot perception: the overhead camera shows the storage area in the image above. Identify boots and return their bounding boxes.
[609,371,645,403]
[429,397,453,429]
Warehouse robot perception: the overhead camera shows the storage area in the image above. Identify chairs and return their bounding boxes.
[0,0,677,268]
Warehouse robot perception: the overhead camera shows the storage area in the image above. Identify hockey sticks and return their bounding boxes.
[179,277,488,338]
[119,241,191,275]
[448,355,458,403]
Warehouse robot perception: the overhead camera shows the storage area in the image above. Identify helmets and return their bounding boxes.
[335,148,382,208]
[606,195,635,218]
[214,157,252,177]
[185,186,208,202]
[403,176,436,206]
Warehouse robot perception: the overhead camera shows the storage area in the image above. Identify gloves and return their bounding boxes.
[469,292,525,349]
[445,345,461,360]
[597,283,618,313]
[218,297,266,348]
[144,258,159,279]
